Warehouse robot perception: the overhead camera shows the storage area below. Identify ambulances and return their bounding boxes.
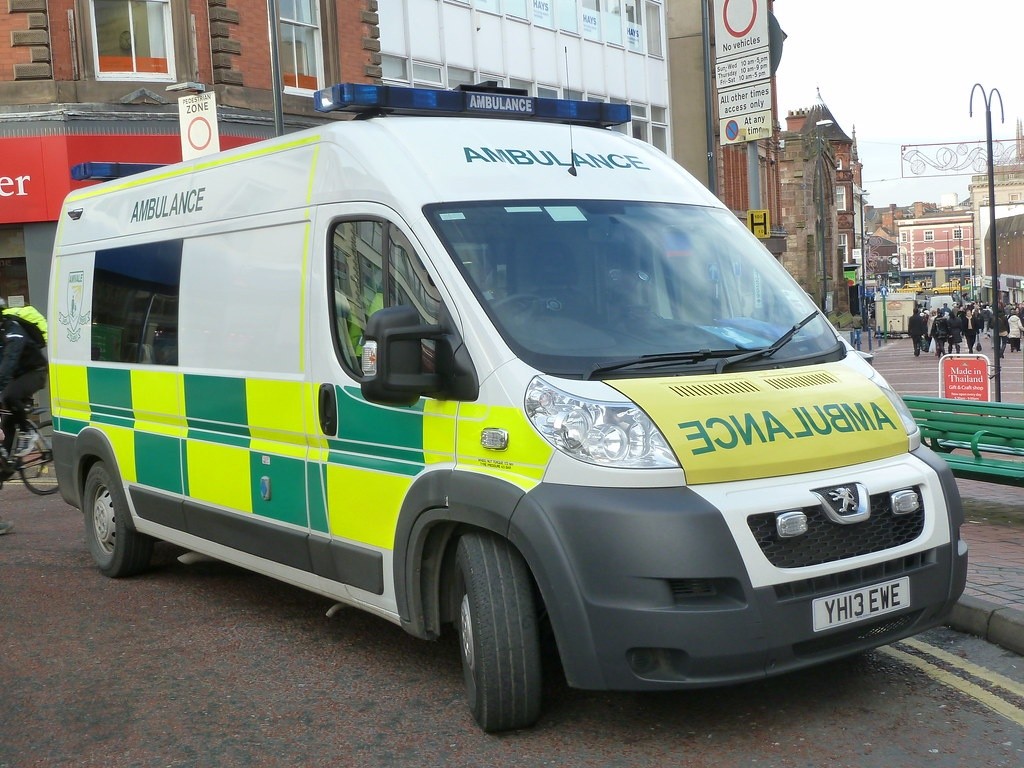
[44,78,972,734]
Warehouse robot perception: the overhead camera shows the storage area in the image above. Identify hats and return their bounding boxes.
[971,301,975,304]
[918,304,923,309]
[1011,311,1018,314]
[943,302,948,306]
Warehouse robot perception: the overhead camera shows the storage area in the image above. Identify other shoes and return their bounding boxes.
[1011,351,1013,352]
[999,351,1004,359]
[0,520,14,534]
[984,333,990,339]
[1017,349,1021,351]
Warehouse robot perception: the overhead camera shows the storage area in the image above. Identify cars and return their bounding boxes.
[901,283,924,295]
[931,281,970,295]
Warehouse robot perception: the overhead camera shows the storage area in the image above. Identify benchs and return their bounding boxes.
[902,394,1024,487]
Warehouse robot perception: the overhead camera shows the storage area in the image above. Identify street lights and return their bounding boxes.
[860,193,871,331]
[815,119,835,321]
[966,211,977,304]
[942,230,951,296]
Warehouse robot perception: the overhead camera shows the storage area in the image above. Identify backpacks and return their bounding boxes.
[0,305,48,349]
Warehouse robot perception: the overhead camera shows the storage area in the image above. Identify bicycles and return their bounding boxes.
[0,404,61,496]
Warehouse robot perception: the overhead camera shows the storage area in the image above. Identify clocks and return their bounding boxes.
[119,30,136,51]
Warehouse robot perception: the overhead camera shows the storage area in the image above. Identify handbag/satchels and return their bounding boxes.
[976,335,982,352]
[999,331,1008,336]
[989,321,994,329]
[920,337,929,352]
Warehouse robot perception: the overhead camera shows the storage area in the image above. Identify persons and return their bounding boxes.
[0,295,47,535]
[852,311,864,344]
[907,292,1024,359]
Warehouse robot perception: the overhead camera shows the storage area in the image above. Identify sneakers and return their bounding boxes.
[14,429,39,457]
